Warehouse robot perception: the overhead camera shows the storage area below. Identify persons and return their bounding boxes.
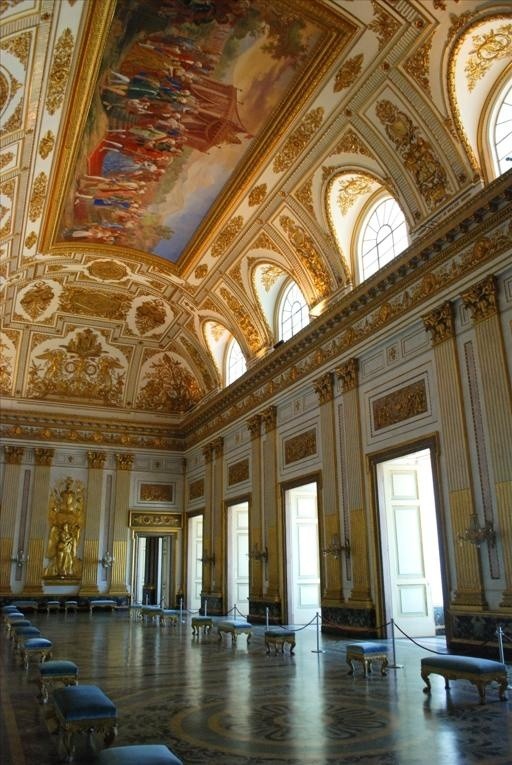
[56,523,80,575]
[60,479,76,514]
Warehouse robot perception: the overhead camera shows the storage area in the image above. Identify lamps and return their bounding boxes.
[457,508,500,547]
[99,550,115,568]
[249,541,268,565]
[10,553,31,567]
[197,548,216,567]
[322,532,351,560]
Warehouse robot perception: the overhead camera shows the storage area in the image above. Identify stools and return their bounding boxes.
[218,620,253,644]
[346,643,388,678]
[39,660,78,703]
[52,684,118,763]
[90,600,181,629]
[265,630,296,656]
[421,656,508,704]
[0,596,76,654]
[100,745,182,764]
[192,615,213,636]
